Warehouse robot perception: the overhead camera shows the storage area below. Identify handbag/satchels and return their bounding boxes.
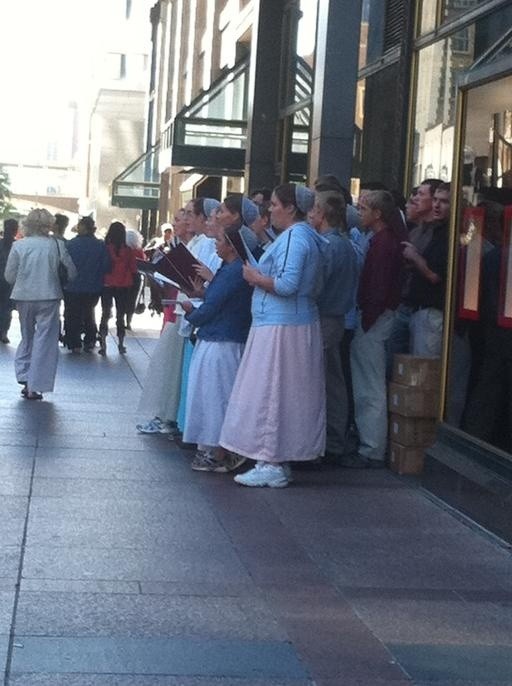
[57,261,68,289]
[135,303,147,314]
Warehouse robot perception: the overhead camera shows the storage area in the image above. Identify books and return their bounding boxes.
[224,225,258,268]
[154,271,183,289]
[155,242,206,292]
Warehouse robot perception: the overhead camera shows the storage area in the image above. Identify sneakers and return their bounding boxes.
[72,345,127,357]
[135,416,386,489]
[22,387,43,401]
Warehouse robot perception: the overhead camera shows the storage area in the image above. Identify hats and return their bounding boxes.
[161,223,174,233]
[295,184,314,213]
[241,197,258,226]
[204,198,220,218]
[239,225,257,252]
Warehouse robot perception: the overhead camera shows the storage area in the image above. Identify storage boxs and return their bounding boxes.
[387,352,440,475]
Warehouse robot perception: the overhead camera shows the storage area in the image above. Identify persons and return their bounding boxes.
[142,222,175,312]
[125,229,145,331]
[4,207,80,399]
[49,213,70,242]
[135,173,450,488]
[0,218,18,344]
[59,216,113,354]
[98,221,138,357]
